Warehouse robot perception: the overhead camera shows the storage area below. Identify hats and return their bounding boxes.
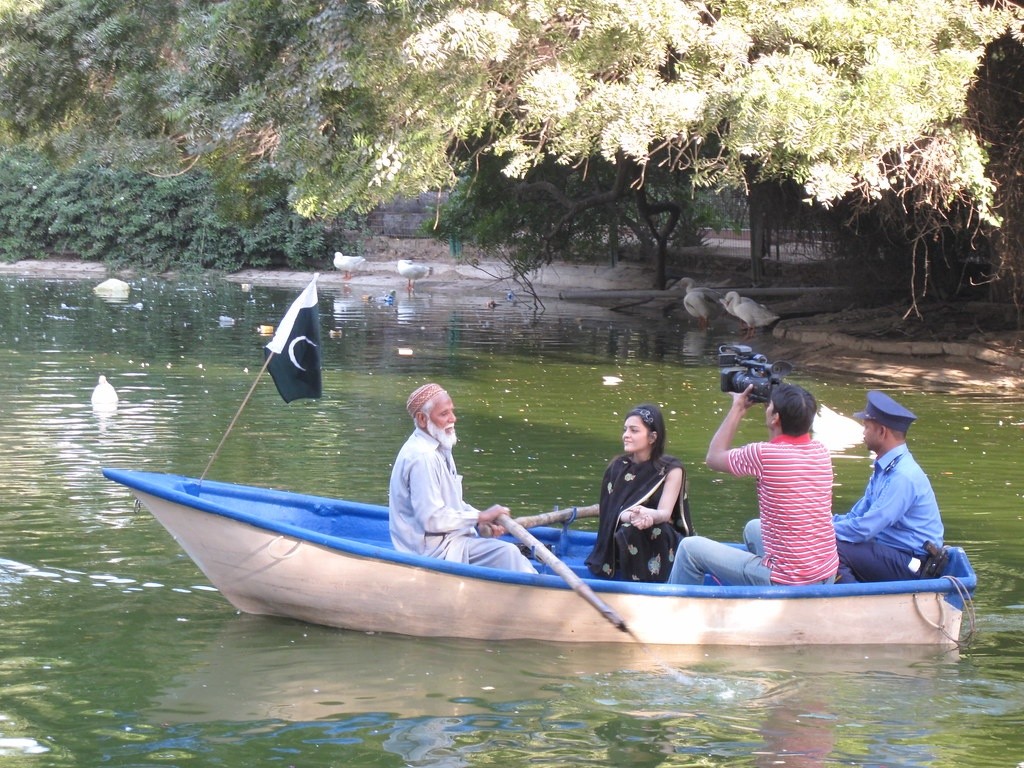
[853,391,917,432]
[407,383,444,418]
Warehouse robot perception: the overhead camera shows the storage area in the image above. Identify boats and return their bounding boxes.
[103,469,989,647]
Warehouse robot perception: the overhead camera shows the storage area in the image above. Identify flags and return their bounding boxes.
[264,277,323,404]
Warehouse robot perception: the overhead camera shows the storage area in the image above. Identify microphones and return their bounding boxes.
[736,351,767,362]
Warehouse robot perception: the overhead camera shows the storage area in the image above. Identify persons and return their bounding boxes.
[583,403,695,584]
[833,390,946,584]
[665,382,840,585]
[388,383,541,574]
